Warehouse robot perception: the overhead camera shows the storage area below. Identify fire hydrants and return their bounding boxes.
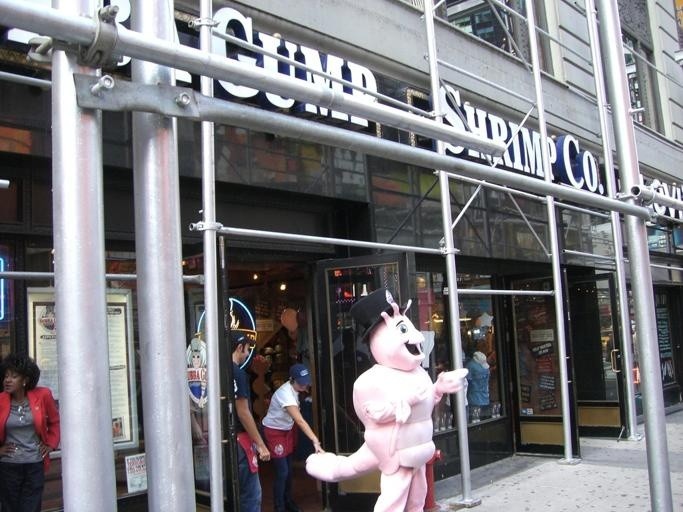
[422,449,443,511]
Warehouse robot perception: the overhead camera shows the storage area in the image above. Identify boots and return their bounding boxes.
[284,483,304,512]
[273,484,286,512]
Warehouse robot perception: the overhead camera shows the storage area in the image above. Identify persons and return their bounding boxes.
[259,363,325,512]
[0,352,60,512]
[229,330,271,512]
[334,327,372,452]
[466,351,490,421]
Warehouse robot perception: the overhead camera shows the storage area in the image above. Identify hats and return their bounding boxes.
[289,363,312,385]
[230,330,256,344]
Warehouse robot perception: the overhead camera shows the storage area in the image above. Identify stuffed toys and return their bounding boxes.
[305,288,469,512]
